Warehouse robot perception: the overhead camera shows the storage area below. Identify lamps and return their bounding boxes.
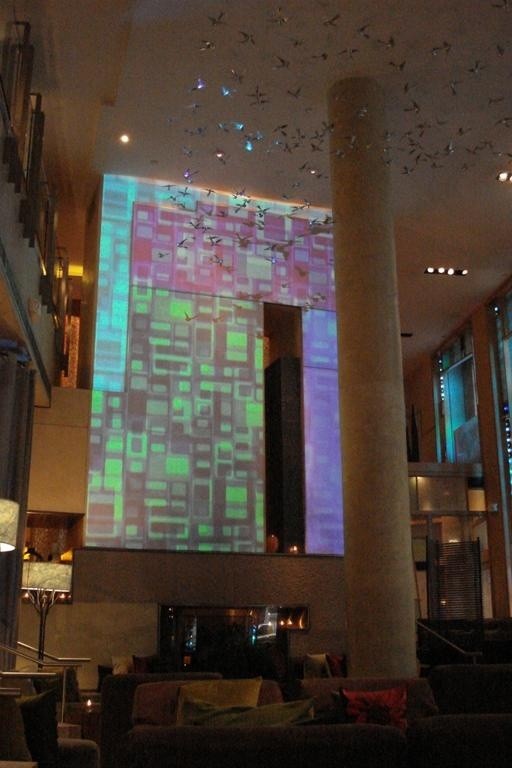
[20,561,72,672]
[0,500,19,552]
[468,486,486,517]
